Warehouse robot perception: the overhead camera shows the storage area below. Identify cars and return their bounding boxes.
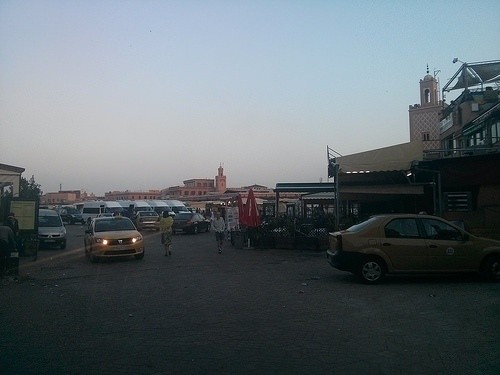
[326,211,500,282]
[136,211,159,229]
[84,216,145,261]
[174,213,211,234]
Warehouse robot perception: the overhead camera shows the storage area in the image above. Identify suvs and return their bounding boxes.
[56,206,82,225]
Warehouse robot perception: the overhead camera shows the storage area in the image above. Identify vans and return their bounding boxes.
[38,208,67,249]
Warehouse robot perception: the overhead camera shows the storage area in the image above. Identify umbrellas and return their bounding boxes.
[243,188,261,250]
[236,194,244,231]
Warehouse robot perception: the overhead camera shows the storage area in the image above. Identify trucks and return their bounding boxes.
[74,203,83,212]
[120,199,191,216]
[81,201,123,223]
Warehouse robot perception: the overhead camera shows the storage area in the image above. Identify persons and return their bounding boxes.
[137,218,143,232]
[160,210,173,257]
[4,213,18,255]
[0,224,16,279]
[196,207,205,217]
[211,212,226,254]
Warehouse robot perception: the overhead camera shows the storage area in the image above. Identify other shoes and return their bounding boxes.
[219,249,222,253]
[169,251,172,256]
